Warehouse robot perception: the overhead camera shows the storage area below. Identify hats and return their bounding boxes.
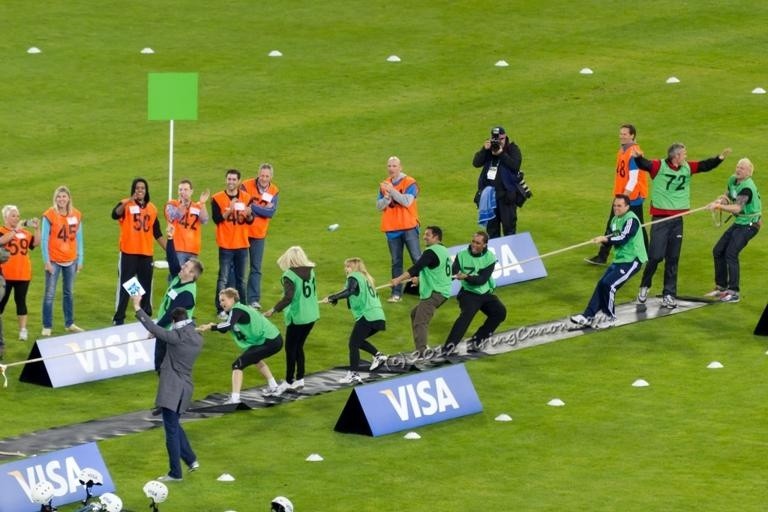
[491,126,506,140]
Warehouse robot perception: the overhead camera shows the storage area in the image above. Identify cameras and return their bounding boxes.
[488,127,504,156]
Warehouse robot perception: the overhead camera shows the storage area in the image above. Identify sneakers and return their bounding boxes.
[157,476,184,481]
[221,397,242,406]
[16,322,86,342]
[262,377,305,397]
[338,369,362,384]
[583,255,608,266]
[662,294,677,309]
[637,286,649,304]
[217,310,229,321]
[368,350,389,372]
[569,312,617,330]
[187,465,200,472]
[385,295,404,303]
[250,301,262,309]
[703,285,741,303]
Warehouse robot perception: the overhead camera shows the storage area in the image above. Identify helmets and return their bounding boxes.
[98,492,124,512]
[142,479,169,504]
[269,495,294,512]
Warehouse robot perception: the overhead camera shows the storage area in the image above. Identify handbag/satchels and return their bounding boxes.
[508,181,528,210]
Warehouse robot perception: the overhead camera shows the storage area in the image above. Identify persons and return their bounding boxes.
[629,144,734,308]
[1,186,84,341]
[376,157,424,303]
[390,226,455,357]
[471,125,521,239]
[570,196,652,327]
[443,231,506,358]
[112,178,210,373]
[210,163,280,319]
[705,158,762,303]
[581,125,649,263]
[131,294,200,483]
[320,257,390,385]
[196,246,320,405]
